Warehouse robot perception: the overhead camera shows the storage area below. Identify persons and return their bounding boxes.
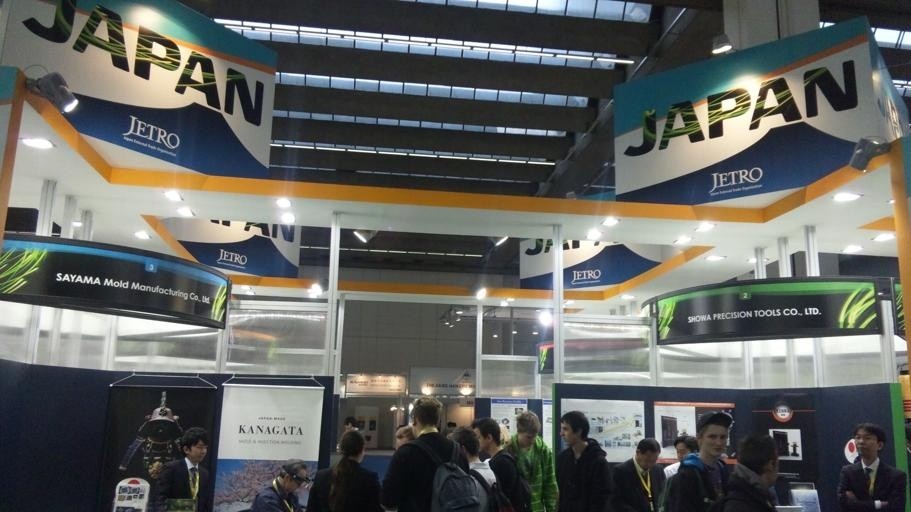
[615,437,667,512]
[837,422,907,512]
[250,459,310,512]
[665,410,735,512]
[380,395,469,512]
[724,434,780,512]
[663,434,700,480]
[305,429,385,512]
[556,411,614,512]
[344,416,358,429]
[471,417,523,512]
[395,425,415,447]
[155,427,210,512]
[502,411,560,512]
[451,425,497,512]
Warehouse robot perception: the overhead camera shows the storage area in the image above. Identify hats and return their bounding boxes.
[697,411,734,429]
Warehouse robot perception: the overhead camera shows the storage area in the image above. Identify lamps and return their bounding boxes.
[748,257,767,266]
[833,191,860,205]
[165,189,183,203]
[41,74,79,114]
[707,254,725,264]
[844,243,863,256]
[871,231,893,244]
[314,279,331,297]
[354,229,379,243]
[851,138,890,172]
[488,236,510,246]
[677,234,691,246]
[695,221,715,234]
[889,198,895,206]
[22,136,54,150]
[712,36,732,56]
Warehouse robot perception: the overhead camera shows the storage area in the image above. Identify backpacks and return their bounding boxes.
[404,438,482,512]
[492,453,532,512]
[705,491,752,512]
[469,468,514,512]
[662,466,706,512]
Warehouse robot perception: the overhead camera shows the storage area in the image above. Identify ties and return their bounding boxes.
[863,466,872,492]
[189,467,197,489]
[640,468,646,484]
